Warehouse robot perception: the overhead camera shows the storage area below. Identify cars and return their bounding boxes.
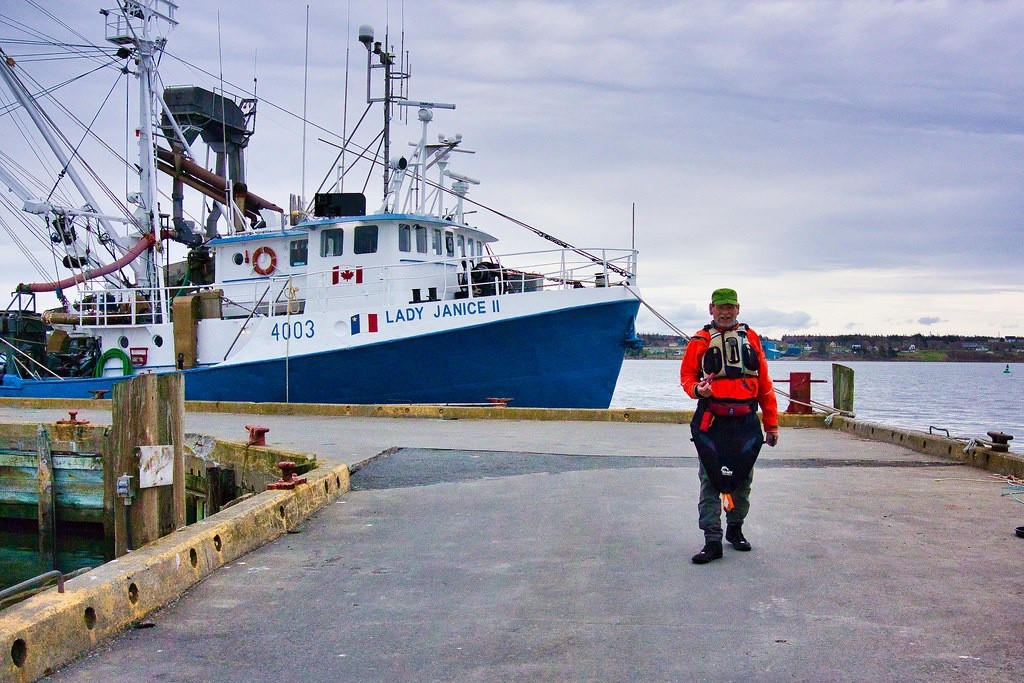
[679,350,683,354]
[674,351,680,355]
[668,340,679,347]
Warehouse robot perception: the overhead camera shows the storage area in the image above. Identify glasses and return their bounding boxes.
[713,305,737,311]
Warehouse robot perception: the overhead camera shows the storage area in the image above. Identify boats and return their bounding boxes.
[1004,370,1010,373]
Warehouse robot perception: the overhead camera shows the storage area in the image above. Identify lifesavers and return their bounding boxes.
[252,246,277,276]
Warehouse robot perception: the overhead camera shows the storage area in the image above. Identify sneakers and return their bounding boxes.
[691,540,723,564]
[726,524,751,551]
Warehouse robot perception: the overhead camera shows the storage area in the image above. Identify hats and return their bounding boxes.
[712,288,737,305]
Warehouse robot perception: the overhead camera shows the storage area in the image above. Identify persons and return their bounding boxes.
[680,289,778,563]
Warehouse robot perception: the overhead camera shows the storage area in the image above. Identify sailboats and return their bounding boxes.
[0,0,642,409]
